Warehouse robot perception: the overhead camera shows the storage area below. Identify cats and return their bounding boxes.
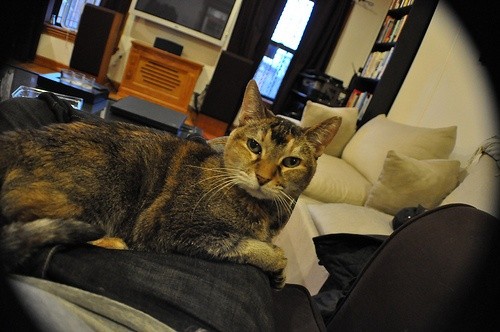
[0,78,343,288]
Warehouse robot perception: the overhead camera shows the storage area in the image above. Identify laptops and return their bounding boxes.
[111,96,188,133]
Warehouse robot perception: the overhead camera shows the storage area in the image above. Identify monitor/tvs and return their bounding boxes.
[129,0,243,48]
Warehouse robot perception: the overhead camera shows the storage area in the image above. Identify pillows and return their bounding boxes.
[298,100,473,212]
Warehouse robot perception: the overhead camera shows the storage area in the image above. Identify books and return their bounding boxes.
[376,15,408,42]
[345,89,373,120]
[360,47,395,80]
[390,0,414,10]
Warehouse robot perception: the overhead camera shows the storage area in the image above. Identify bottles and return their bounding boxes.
[49,15,56,25]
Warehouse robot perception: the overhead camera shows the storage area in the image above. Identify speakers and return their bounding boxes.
[70,3,124,84]
[200,50,254,123]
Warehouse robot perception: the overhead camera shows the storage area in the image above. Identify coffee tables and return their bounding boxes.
[1,63,203,138]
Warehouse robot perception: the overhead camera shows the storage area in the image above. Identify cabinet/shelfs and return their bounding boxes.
[340,0,438,128]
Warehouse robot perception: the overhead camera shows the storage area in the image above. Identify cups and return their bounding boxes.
[81,76,96,91]
[71,72,86,86]
[61,68,74,84]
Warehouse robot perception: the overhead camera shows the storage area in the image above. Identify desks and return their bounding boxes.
[110,42,203,124]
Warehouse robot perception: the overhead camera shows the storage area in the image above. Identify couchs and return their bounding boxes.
[257,112,500,296]
[272,201,500,332]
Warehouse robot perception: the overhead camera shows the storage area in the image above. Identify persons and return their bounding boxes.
[0,92,274,332]
